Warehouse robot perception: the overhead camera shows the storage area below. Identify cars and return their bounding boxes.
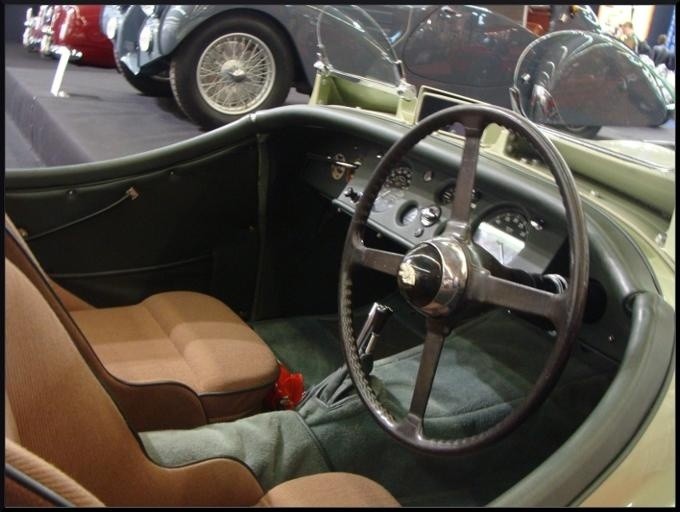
[18,4,118,69]
[102,3,674,140]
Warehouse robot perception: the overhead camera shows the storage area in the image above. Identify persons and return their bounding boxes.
[648,33,667,67]
[617,22,641,55]
[638,35,653,62]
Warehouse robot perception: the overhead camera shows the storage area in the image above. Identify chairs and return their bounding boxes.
[4,207,282,431]
[4,257,405,507]
